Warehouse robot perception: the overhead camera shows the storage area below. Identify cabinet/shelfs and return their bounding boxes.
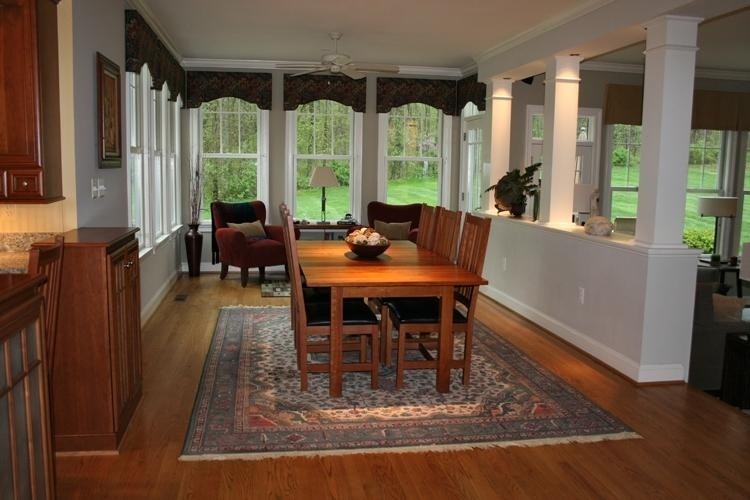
[0,270,60,499]
[29,222,148,461]
[0,0,73,208]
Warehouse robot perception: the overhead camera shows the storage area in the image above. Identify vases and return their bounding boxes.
[183,221,205,279]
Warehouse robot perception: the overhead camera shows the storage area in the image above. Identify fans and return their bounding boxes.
[277,29,401,84]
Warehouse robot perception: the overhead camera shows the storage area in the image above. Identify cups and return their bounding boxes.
[711,254,720,265]
[731,257,737,265]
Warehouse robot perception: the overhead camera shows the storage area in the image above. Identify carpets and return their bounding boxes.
[174,305,644,466]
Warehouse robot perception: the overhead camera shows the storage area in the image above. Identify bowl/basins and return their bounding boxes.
[344,235,390,257]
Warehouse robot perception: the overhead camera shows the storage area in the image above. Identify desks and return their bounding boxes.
[696,261,744,299]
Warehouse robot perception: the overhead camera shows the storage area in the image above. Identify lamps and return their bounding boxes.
[695,195,741,265]
[306,157,344,225]
[573,183,596,227]
[737,241,750,283]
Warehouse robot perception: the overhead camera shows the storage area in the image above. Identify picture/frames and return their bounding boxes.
[94,49,125,170]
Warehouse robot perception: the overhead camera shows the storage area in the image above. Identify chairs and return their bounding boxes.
[274,200,497,397]
[26,233,68,391]
[207,196,300,290]
[692,281,750,393]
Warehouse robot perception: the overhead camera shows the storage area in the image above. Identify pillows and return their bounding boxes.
[222,216,269,241]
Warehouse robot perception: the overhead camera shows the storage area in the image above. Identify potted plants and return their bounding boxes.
[484,159,544,219]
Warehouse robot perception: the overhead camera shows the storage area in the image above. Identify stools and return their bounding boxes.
[717,330,750,412]
